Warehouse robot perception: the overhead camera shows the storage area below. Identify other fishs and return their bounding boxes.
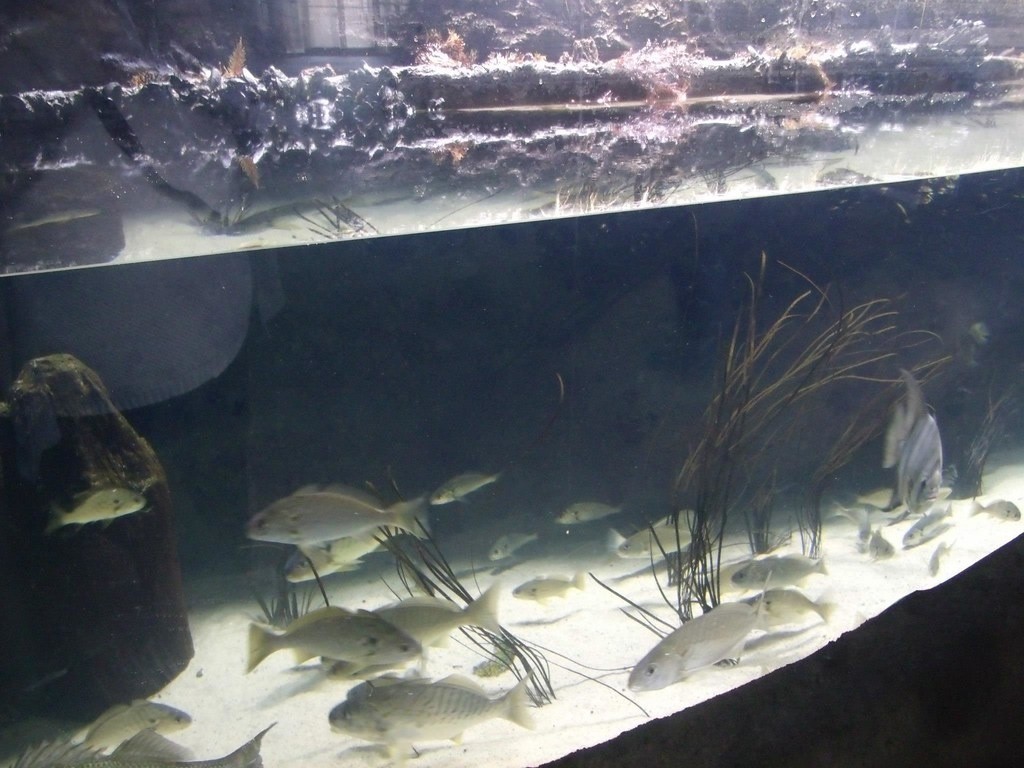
[47,487,145,534]
[8,697,278,768]
[246,321,1023,768]
